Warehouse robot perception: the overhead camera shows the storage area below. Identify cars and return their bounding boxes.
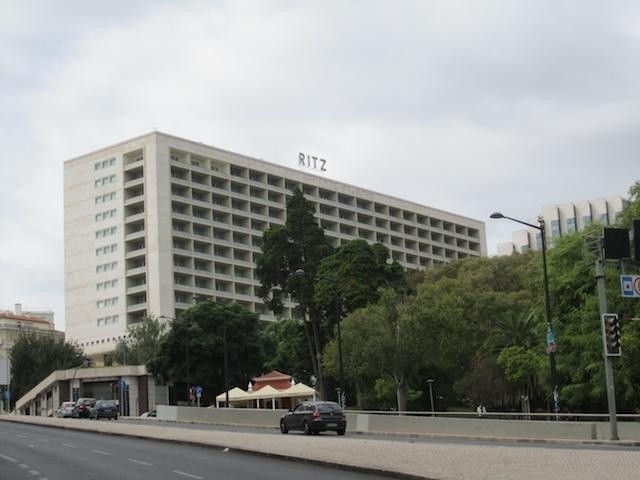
[54,397,118,420]
[280,400,348,435]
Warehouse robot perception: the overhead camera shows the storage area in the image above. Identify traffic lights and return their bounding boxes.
[601,313,623,358]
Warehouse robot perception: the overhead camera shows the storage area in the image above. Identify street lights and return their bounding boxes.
[295,269,348,409]
[192,293,229,409]
[335,388,342,407]
[426,379,436,416]
[489,212,562,421]
[70,361,96,402]
[159,315,191,406]
